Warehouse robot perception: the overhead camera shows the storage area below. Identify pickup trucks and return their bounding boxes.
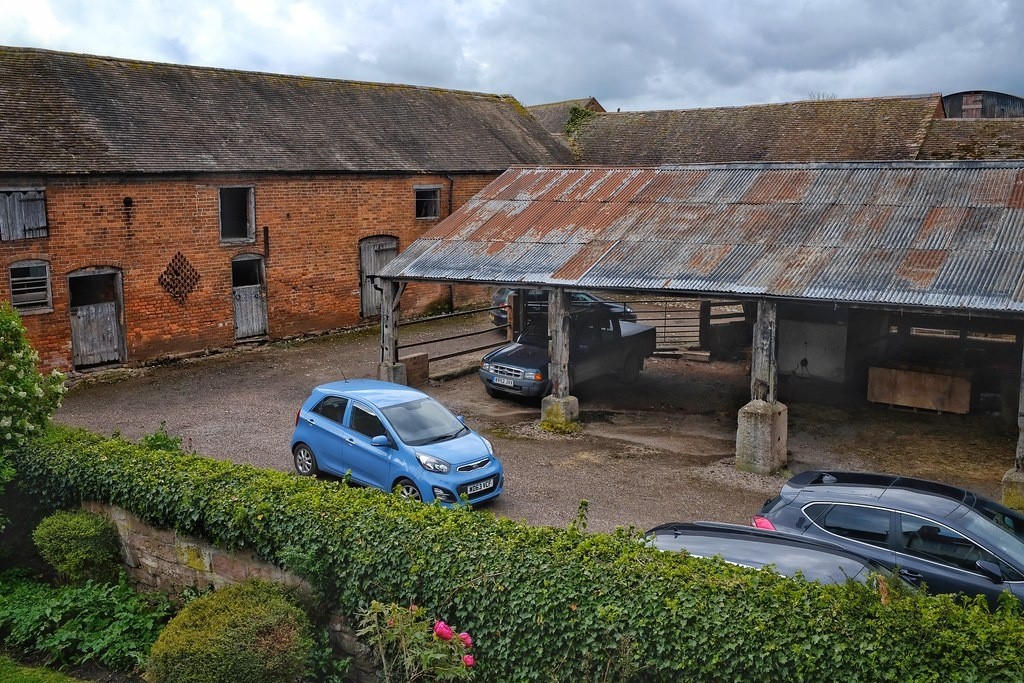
[478,305,656,403]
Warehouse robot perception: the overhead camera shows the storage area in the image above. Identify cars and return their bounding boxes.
[291,378,504,509]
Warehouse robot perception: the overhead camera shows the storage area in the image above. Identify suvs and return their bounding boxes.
[642,520,946,618]
[750,469,1024,607]
[486,285,639,336]
